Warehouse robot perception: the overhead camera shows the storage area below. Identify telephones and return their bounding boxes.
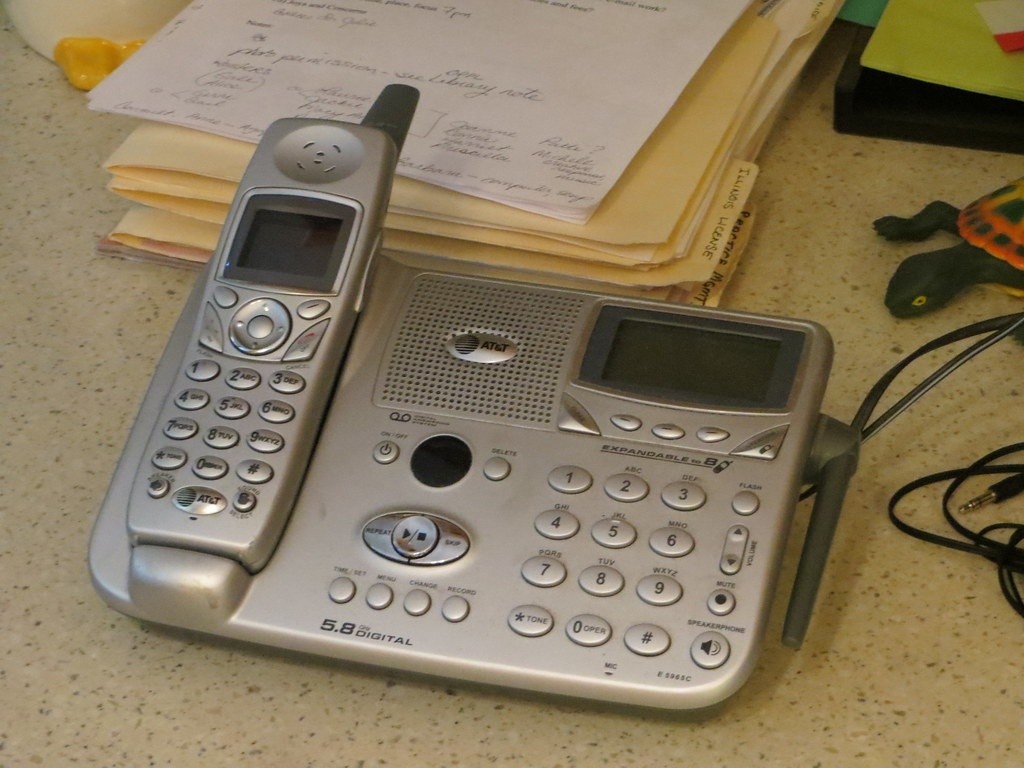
[88,84,862,713]
